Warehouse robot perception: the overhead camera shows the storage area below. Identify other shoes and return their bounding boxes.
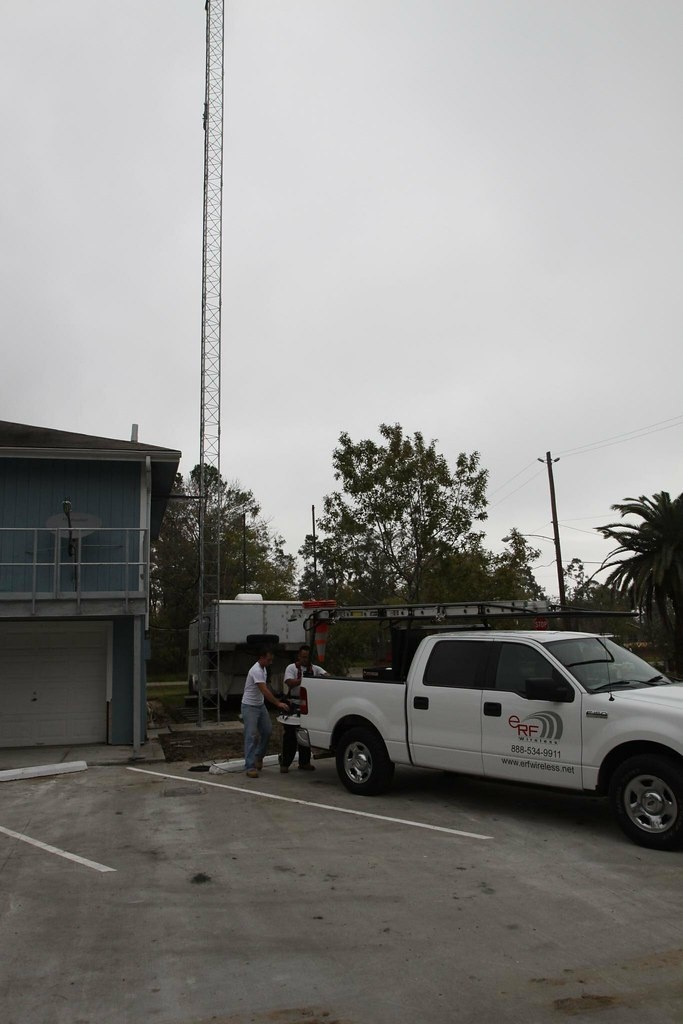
[255,757,262,769]
[281,766,287,773]
[299,764,315,770]
[247,769,257,778]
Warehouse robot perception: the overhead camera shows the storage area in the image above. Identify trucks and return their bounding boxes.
[186,591,337,711]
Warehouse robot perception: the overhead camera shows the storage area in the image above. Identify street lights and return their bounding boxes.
[502,533,571,612]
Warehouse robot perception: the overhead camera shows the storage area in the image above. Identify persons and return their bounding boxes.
[279,645,331,773]
[241,648,289,778]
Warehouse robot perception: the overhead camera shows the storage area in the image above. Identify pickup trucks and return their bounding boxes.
[274,626,683,852]
